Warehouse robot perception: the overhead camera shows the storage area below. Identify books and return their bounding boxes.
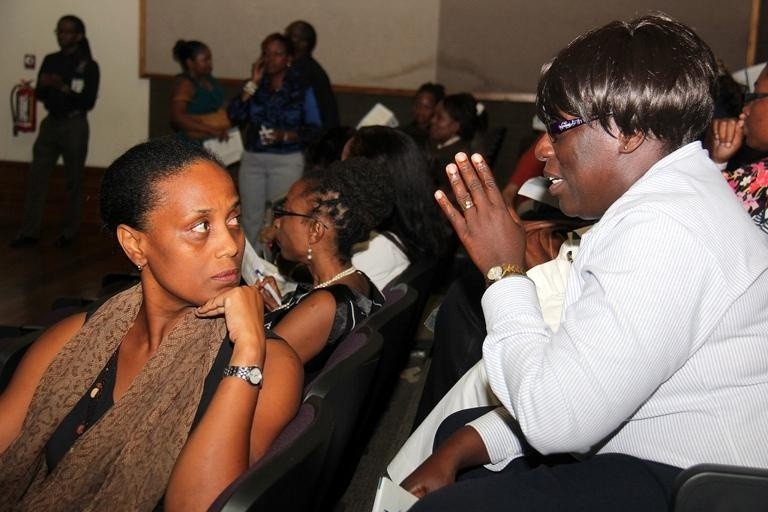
[368,475,421,511]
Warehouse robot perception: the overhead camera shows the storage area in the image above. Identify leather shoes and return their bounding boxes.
[9,234,70,250]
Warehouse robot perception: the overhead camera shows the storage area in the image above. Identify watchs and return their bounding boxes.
[220,360,264,392]
[483,256,529,289]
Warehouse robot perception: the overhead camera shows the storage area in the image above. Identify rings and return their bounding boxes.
[458,197,474,214]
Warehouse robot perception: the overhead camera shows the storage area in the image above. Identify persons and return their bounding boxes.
[0,130,305,511]
[384,12,768,511]
[10,13,101,251]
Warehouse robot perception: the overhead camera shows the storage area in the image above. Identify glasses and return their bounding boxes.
[742,88,768,105]
[542,106,627,144]
[270,199,328,233]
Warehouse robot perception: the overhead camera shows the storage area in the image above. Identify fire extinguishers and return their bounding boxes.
[10,79,37,137]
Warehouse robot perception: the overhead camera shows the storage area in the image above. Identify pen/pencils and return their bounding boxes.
[255,269,283,307]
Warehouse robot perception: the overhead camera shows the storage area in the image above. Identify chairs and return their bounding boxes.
[208,390,336,512]
[357,283,418,326]
[380,255,440,296]
[309,324,383,495]
[669,459,768,512]
